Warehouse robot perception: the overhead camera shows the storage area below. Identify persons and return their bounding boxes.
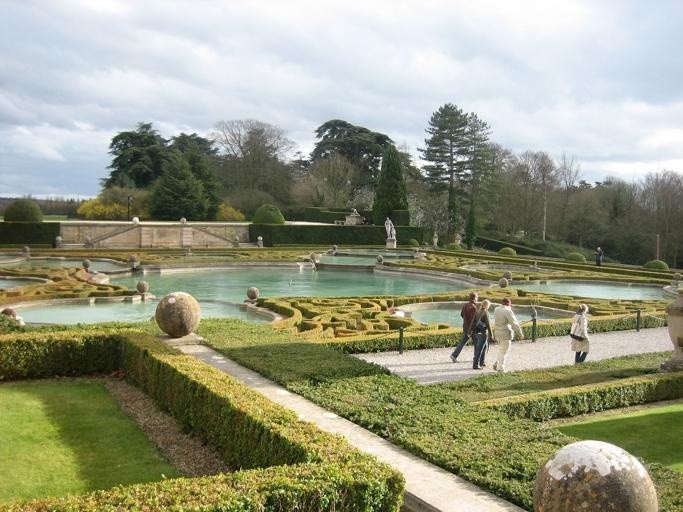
[390,225,395,238]
[467,299,494,370]
[492,297,524,373]
[594,246,604,266]
[384,217,393,238]
[570,303,591,364]
[449,291,478,364]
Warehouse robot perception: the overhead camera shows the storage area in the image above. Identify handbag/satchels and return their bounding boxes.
[476,321,486,331]
[570,323,584,340]
